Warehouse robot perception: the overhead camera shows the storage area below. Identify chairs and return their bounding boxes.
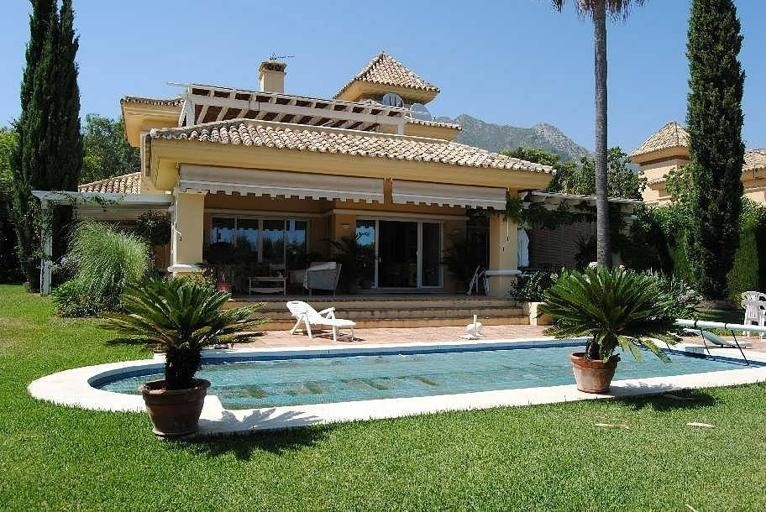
[740,290,766,341]
[684,328,753,349]
[285,300,356,343]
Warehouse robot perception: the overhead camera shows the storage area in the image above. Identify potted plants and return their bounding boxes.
[95,270,270,434]
[217,264,233,293]
[531,263,704,393]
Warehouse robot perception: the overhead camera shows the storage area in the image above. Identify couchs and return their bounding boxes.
[288,260,343,299]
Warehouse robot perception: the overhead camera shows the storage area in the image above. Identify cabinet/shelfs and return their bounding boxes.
[248,276,288,296]
